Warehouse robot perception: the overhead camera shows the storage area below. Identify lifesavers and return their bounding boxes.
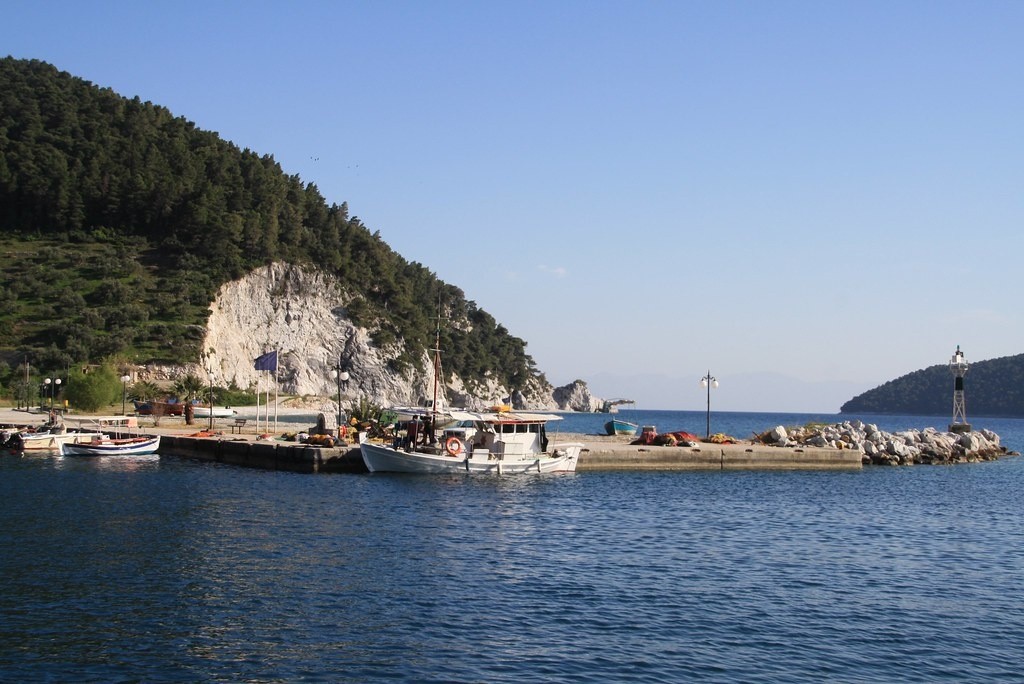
[446,436,462,455]
[100,419,109,428]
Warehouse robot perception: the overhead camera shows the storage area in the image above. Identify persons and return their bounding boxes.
[422,411,434,447]
[405,415,418,453]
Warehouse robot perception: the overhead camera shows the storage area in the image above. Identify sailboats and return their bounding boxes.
[354,292,574,477]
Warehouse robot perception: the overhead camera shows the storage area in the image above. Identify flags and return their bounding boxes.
[254,350,276,371]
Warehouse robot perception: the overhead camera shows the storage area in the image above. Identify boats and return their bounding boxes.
[0,420,105,450]
[603,416,638,436]
[60,420,161,457]
[442,393,586,472]
[182,404,234,418]
[131,398,185,416]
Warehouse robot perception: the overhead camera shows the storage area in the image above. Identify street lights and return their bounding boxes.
[44,373,62,411]
[699,368,719,438]
[329,360,350,426]
[206,364,216,431]
[120,369,131,415]
